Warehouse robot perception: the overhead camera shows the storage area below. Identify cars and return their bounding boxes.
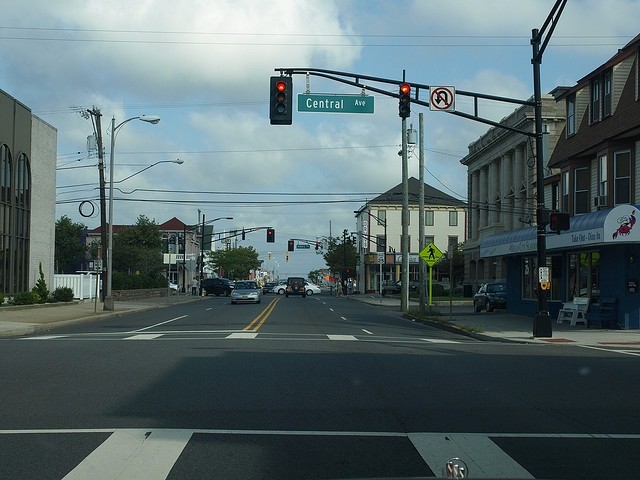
[264,283,276,295]
[274,281,320,296]
[392,281,417,293]
[170,283,179,295]
[474,282,506,313]
[231,280,261,304]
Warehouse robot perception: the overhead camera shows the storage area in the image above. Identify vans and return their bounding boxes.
[200,278,236,296]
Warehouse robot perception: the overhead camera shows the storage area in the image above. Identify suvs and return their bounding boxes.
[286,277,305,298]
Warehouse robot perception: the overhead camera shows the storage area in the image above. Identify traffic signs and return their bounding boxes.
[297,93,374,113]
[296,244,310,249]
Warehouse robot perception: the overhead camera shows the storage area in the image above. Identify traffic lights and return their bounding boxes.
[399,84,410,117]
[316,242,318,249]
[285,253,289,261]
[353,236,357,244]
[270,75,291,125]
[288,240,294,252]
[267,228,275,242]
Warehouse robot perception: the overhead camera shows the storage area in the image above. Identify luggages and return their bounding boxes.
[533,310,552,337]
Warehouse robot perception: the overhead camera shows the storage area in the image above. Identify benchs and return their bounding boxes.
[584,297,620,329]
[557,296,590,327]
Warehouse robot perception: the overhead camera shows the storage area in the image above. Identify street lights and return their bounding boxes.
[202,214,233,295]
[354,210,387,294]
[103,114,185,311]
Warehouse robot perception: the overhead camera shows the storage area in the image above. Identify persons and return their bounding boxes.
[190,277,199,296]
[333,282,336,295]
[348,282,353,294]
[389,246,392,252]
[344,281,348,295]
[336,282,345,298]
